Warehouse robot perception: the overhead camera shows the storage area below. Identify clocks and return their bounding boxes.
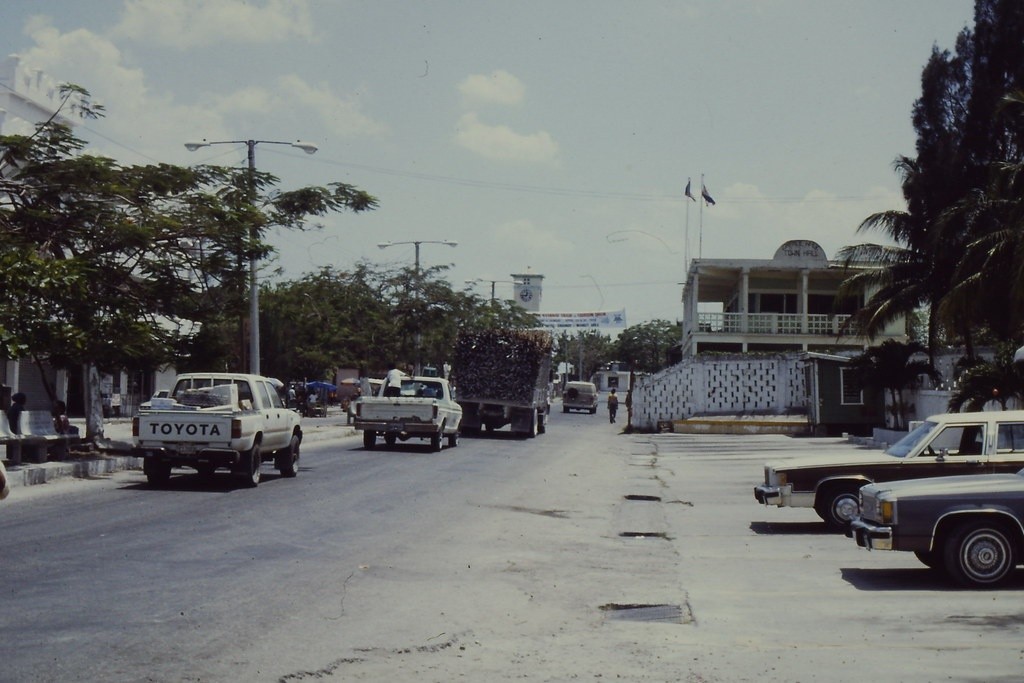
[520,288,533,302]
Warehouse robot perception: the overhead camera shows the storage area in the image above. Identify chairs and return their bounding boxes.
[958,441,983,455]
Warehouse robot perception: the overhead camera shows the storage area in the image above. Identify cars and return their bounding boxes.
[846,469,1024,590]
[138,387,169,415]
[753,410,1024,535]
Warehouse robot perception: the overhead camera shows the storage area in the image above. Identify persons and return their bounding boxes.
[8,394,28,435]
[57,400,80,434]
[288,384,318,408]
[0,460,10,500]
[607,389,619,424]
[384,363,409,397]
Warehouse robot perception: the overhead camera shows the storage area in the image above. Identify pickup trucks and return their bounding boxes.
[355,375,463,452]
[132,372,304,488]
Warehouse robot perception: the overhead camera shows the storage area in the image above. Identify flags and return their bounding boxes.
[685,181,690,197]
[702,185,716,205]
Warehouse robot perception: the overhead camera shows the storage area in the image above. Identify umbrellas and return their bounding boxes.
[308,381,336,392]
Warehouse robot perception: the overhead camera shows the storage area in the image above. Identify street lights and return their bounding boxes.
[184,139,319,374]
[463,279,525,306]
[377,239,459,375]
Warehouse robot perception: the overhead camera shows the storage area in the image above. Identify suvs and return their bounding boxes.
[562,381,599,414]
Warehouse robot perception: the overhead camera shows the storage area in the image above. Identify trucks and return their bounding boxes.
[452,354,553,439]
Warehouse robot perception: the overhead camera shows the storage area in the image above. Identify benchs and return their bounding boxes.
[0,410,80,465]
